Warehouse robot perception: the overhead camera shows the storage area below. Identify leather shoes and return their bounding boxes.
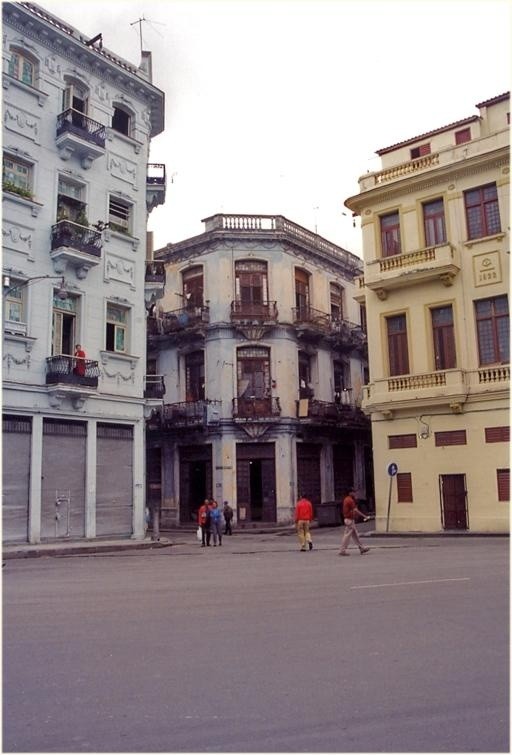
[339,551,350,555]
[200,543,222,547]
[360,548,369,554]
[300,542,312,551]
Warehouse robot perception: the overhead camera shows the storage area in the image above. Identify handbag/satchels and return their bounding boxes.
[197,526,203,541]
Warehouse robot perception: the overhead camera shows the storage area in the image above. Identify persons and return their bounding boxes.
[197,498,233,547]
[73,344,86,377]
[341,487,370,556]
[294,491,313,551]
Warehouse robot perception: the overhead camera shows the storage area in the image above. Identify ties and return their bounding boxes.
[205,505,208,513]
